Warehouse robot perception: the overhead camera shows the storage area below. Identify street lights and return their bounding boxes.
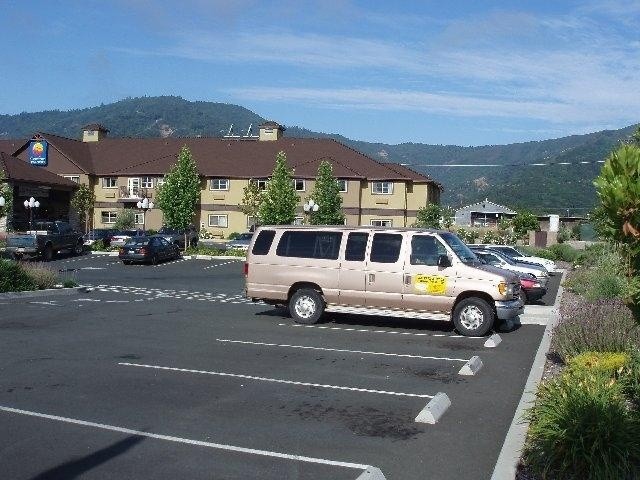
[0,194,5,219]
[136,196,154,230]
[23,195,41,232]
[303,199,319,225]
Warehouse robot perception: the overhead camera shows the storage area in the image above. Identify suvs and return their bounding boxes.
[148,224,200,249]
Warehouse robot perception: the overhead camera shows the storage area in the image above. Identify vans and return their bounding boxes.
[241,224,526,336]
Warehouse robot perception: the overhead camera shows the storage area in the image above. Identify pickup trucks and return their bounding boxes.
[0,219,85,261]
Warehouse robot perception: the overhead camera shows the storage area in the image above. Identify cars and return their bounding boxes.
[81,227,120,248]
[117,236,181,266]
[438,245,558,302]
[226,232,255,252]
[110,228,146,249]
